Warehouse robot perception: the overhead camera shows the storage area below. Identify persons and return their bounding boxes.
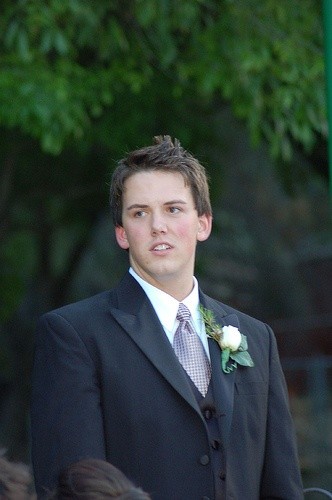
[48,457,151,500]
[31,136,307,500]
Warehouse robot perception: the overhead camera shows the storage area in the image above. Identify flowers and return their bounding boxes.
[197,303,257,375]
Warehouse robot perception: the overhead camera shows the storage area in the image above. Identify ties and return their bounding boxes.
[173,303,211,397]
